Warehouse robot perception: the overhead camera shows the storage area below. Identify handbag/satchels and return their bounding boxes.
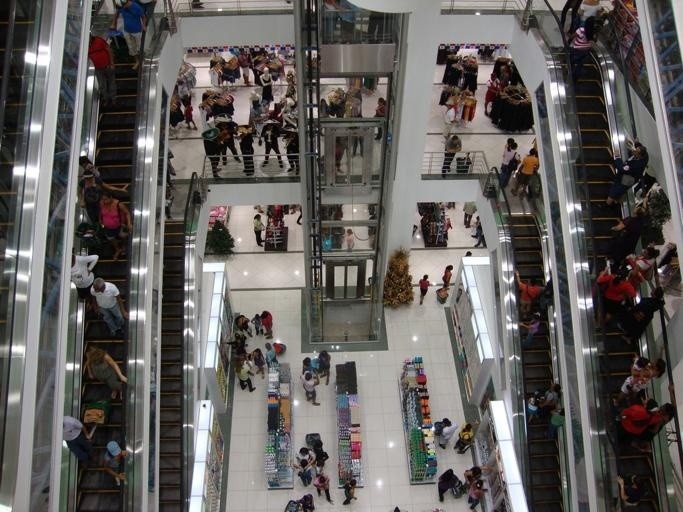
[507,152,520,170]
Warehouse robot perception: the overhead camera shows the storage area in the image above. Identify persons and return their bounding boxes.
[443,265,453,287]
[322,2,384,45]
[438,418,497,511]
[300,350,331,405]
[292,439,334,502]
[225,311,278,392]
[343,479,357,505]
[419,274,430,305]
[64,2,159,490]
[297,493,315,511]
[171,49,540,246]
[191,1,204,9]
[555,1,673,510]
[466,251,472,256]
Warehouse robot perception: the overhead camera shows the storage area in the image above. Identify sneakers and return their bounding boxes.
[287,168,293,172]
[243,170,254,176]
[263,161,268,167]
[214,167,221,172]
[106,440,122,457]
[250,387,256,392]
[511,189,525,200]
[313,402,320,406]
[295,170,299,175]
[242,384,247,390]
[306,397,312,401]
[222,160,227,165]
[235,158,242,163]
[258,239,265,247]
[297,221,302,226]
[279,163,283,169]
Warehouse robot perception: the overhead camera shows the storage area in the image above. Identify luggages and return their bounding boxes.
[81,400,112,442]
